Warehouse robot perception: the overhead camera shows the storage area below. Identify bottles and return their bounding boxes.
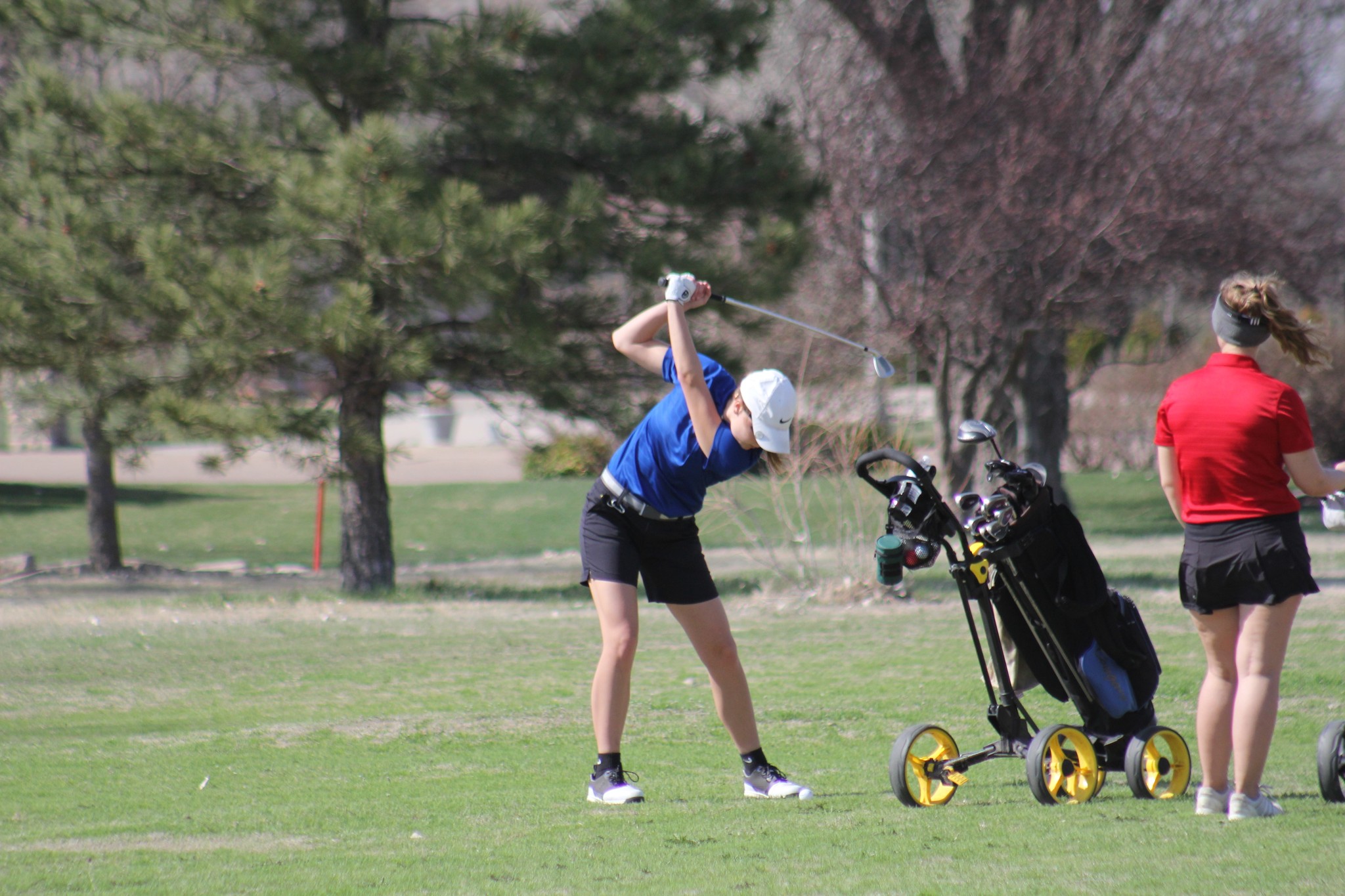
[890,455,931,516]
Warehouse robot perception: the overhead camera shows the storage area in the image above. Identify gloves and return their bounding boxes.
[665,273,697,306]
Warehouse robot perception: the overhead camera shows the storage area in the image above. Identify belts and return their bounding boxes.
[600,467,693,521]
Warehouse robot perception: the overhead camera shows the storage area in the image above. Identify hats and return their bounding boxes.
[740,368,796,454]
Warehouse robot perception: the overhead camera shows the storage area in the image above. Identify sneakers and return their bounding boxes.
[1195,785,1233,814]
[743,763,803,799]
[1227,785,1283,821]
[586,764,644,804]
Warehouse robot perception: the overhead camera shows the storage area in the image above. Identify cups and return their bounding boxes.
[876,534,902,586]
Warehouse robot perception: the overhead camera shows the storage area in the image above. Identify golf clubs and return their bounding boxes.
[657,273,900,381]
[953,418,1049,545]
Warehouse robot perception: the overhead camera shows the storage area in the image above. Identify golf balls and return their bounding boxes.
[798,788,813,801]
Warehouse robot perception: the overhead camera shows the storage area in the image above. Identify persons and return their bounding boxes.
[575,271,815,806]
[1153,270,1345,823]
